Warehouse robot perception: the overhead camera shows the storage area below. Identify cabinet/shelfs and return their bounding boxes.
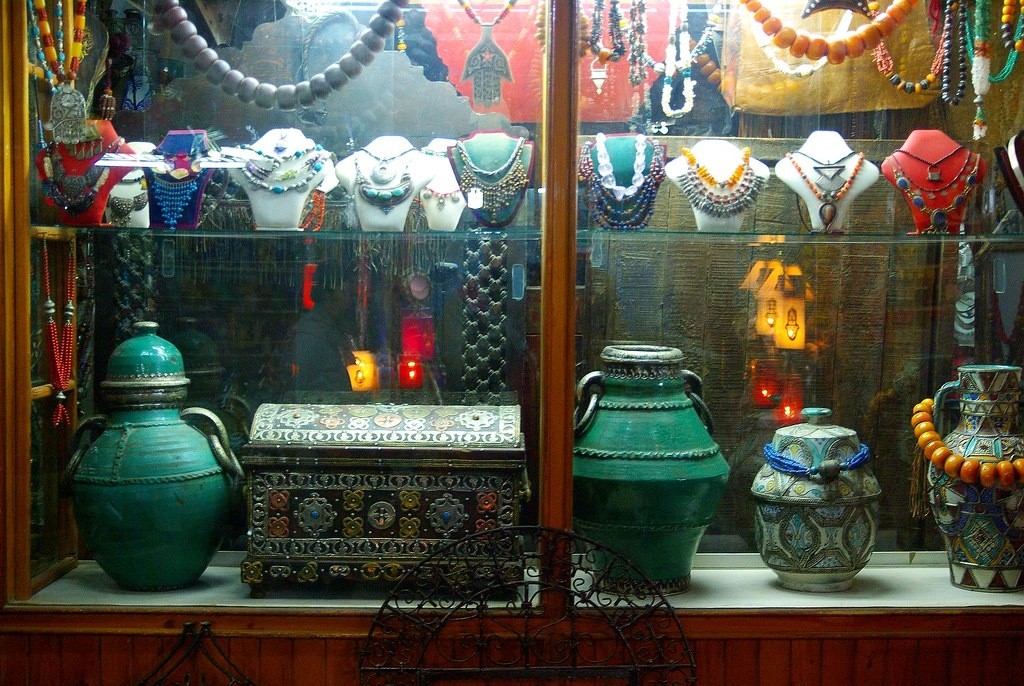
[0,0,1024,686]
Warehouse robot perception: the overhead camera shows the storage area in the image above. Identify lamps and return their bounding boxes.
[740,234,815,424]
[345,302,436,392]
